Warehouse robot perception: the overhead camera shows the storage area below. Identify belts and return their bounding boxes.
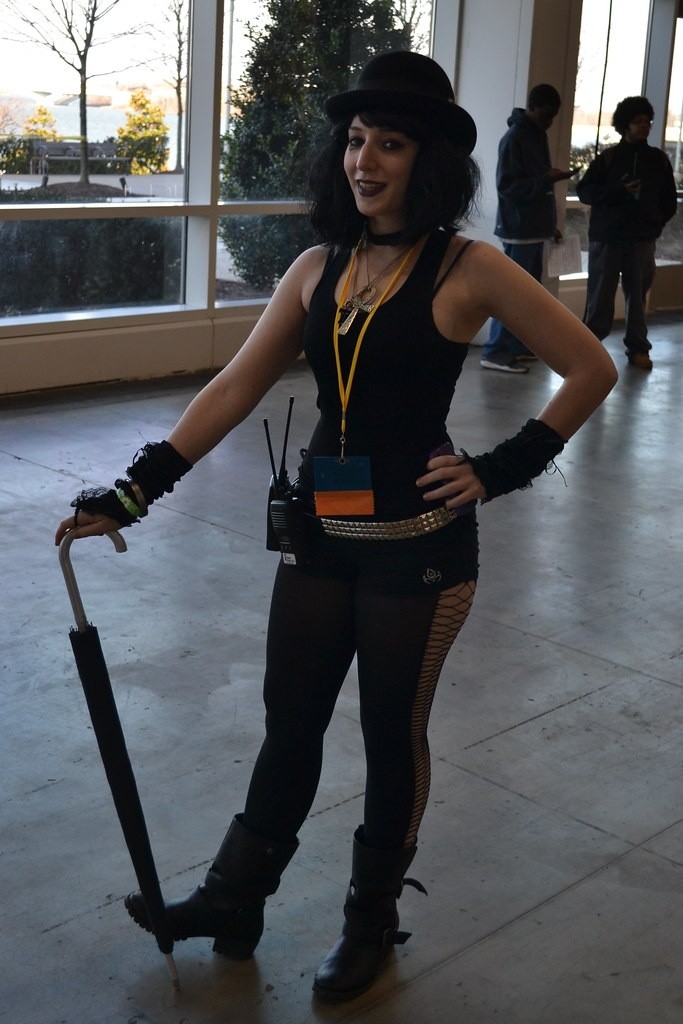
[296,493,477,541]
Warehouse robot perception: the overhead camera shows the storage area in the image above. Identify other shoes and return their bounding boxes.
[628,353,653,369]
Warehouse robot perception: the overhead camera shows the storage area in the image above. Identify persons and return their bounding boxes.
[478,83,570,376]
[50,48,621,1014]
[575,95,678,372]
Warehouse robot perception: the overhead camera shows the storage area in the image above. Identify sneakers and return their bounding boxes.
[509,349,538,360]
[480,349,530,372]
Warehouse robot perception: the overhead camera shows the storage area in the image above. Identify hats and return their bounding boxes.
[323,50,476,155]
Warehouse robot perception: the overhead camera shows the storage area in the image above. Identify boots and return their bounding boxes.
[313,823,428,1004]
[126,813,299,961]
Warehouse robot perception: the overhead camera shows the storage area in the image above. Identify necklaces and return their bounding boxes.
[336,228,415,336]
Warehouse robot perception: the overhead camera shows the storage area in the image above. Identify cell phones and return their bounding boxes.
[566,168,580,175]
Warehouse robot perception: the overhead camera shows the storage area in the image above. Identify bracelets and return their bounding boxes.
[115,477,148,519]
